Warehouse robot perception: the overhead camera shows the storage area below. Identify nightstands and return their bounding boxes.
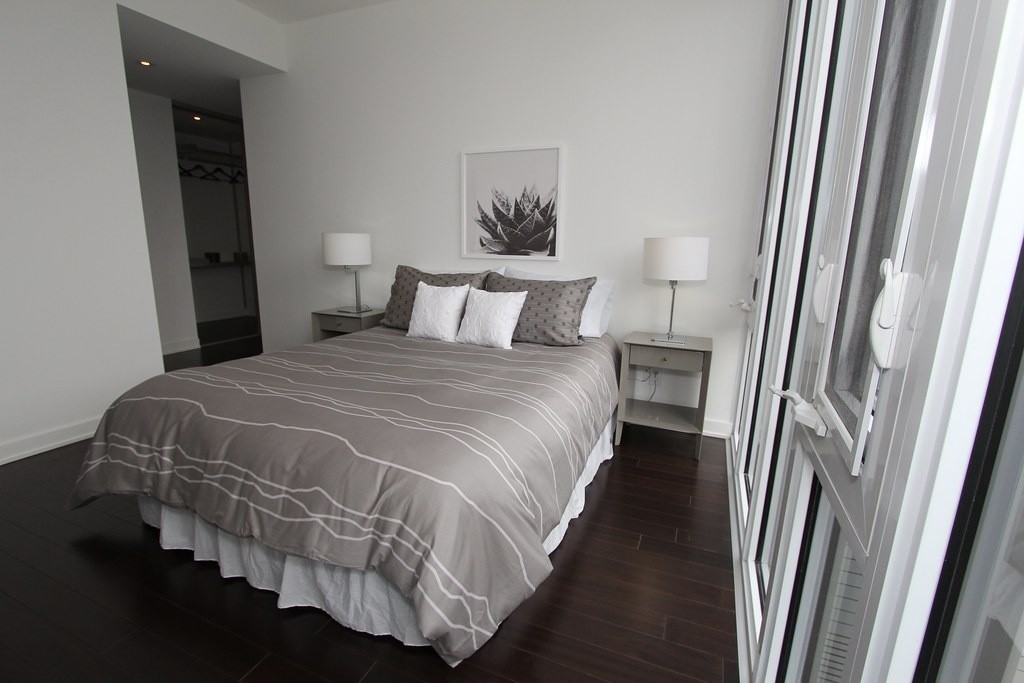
[615,330,713,461]
[311,306,386,342]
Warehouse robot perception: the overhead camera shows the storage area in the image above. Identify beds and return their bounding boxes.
[64,333,623,670]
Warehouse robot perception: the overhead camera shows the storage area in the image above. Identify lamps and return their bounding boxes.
[643,236,710,344]
[322,231,372,315]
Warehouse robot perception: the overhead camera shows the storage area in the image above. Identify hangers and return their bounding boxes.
[177,157,248,183]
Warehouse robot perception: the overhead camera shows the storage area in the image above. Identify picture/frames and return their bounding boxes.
[460,144,564,260]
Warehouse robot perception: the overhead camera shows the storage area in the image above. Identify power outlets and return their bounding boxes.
[643,367,662,385]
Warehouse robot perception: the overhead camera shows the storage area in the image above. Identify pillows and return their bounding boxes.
[378,265,616,350]
